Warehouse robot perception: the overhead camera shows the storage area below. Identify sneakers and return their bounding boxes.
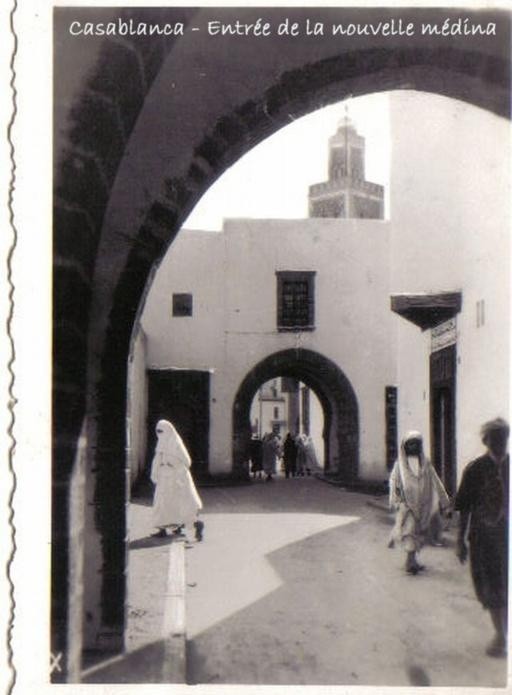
[485,630,508,655]
[151,521,203,541]
[406,565,425,575]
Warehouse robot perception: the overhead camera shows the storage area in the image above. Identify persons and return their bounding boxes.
[450,416,509,662]
[147,416,204,541]
[246,430,321,482]
[385,428,454,576]
